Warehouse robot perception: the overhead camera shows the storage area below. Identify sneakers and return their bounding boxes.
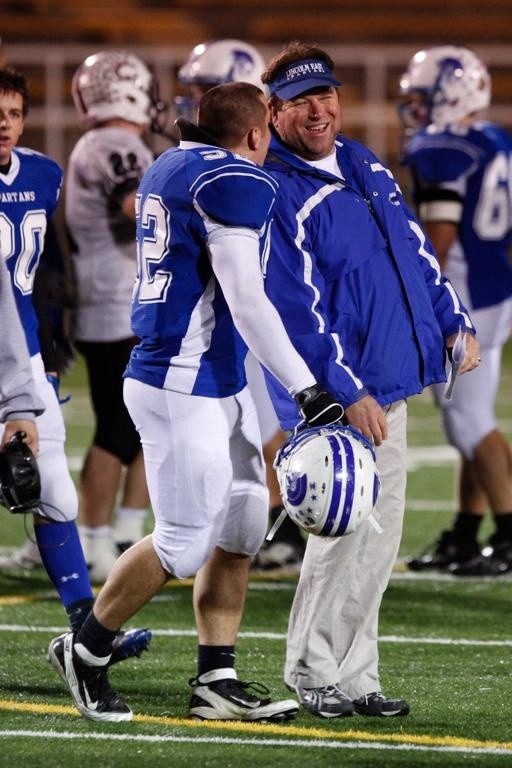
[1,530,51,583]
[45,633,134,723]
[80,535,122,586]
[188,666,301,725]
[447,534,512,575]
[353,691,410,718]
[111,517,144,556]
[283,682,355,719]
[404,529,481,571]
[255,534,306,573]
[109,628,152,665]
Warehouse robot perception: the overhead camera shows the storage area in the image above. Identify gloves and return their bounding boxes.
[294,381,350,429]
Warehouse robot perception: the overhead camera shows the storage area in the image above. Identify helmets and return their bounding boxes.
[171,37,272,121]
[69,47,170,134]
[272,424,381,538]
[395,43,492,135]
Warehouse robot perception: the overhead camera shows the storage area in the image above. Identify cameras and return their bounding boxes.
[1,442,42,511]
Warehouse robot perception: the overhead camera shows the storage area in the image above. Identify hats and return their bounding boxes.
[265,55,343,102]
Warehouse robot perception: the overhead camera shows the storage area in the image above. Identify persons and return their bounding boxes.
[0,70,152,659]
[182,40,266,134]
[0,269,38,457]
[47,83,342,723]
[60,50,157,583]
[382,47,512,575]
[1,253,47,510]
[265,47,478,718]
